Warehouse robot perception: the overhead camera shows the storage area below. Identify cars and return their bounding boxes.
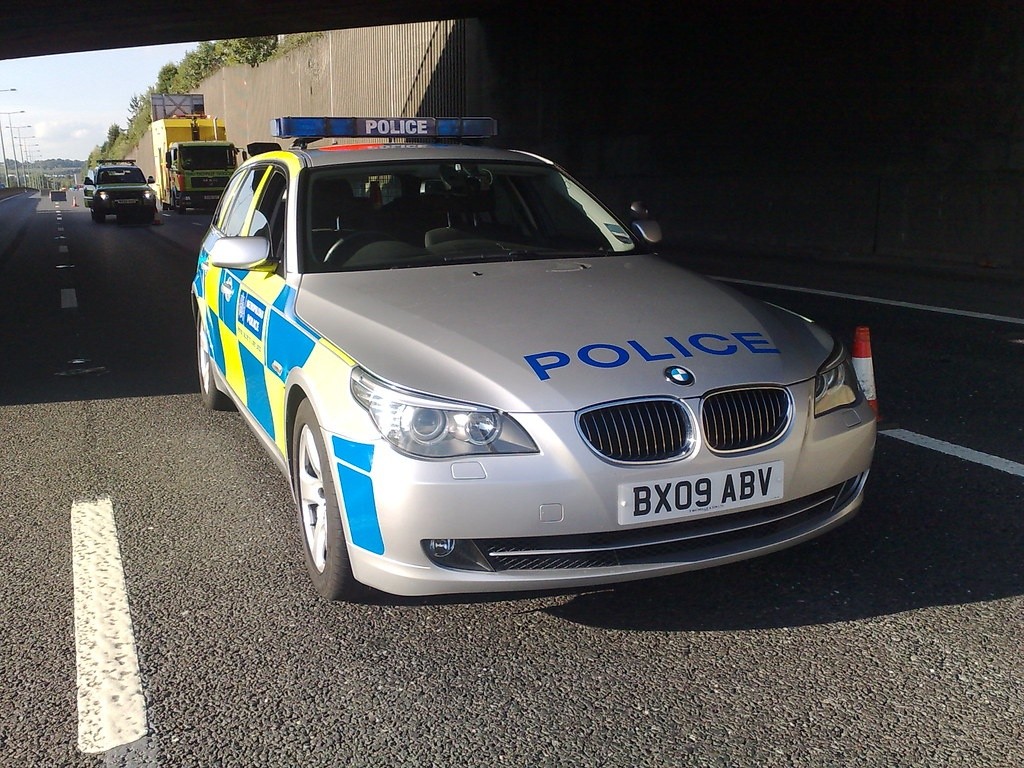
[189,116,879,604]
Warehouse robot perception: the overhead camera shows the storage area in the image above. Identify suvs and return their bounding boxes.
[82,159,157,224]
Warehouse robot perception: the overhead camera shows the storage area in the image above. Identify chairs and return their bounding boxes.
[424,197,522,248]
[380,177,440,215]
[313,180,357,229]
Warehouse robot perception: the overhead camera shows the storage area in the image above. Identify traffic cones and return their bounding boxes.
[71,195,80,208]
[849,325,900,430]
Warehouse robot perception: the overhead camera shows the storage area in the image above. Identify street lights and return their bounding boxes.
[0,88,43,187]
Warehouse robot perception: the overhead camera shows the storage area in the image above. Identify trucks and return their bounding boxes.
[150,113,248,215]
